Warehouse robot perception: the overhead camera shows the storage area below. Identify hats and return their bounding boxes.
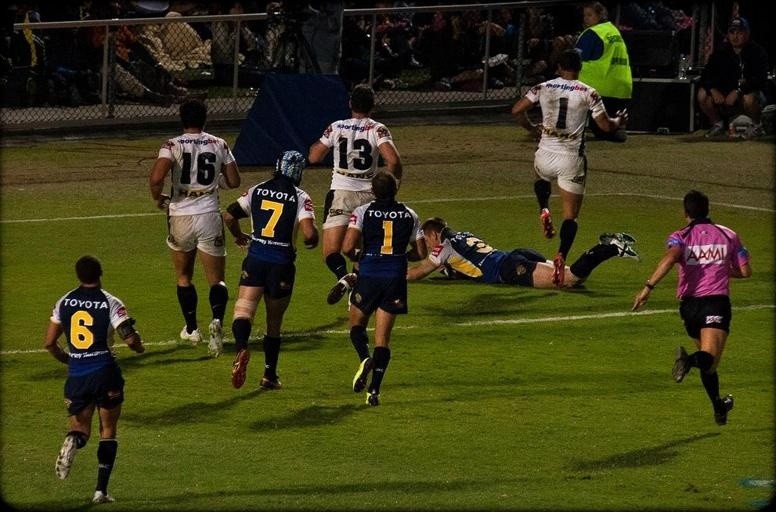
[726,17,747,31]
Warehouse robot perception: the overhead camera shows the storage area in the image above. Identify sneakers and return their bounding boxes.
[551,254,566,289]
[365,393,379,406]
[54,434,76,480]
[704,123,721,139]
[207,318,223,360]
[327,273,360,305]
[610,233,639,258]
[352,357,373,393]
[599,232,636,246]
[539,208,557,239]
[92,490,116,505]
[179,324,202,341]
[672,344,690,384]
[259,376,282,390]
[713,392,734,426]
[231,348,251,389]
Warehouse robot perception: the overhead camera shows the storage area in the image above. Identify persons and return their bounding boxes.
[558,3,633,143]
[266,1,302,70]
[404,217,639,288]
[631,191,752,425]
[210,5,257,83]
[512,47,628,287]
[223,150,319,388]
[150,101,242,357]
[335,0,548,91]
[309,85,403,311]
[698,18,766,139]
[44,256,144,503]
[342,173,418,407]
[0,0,214,108]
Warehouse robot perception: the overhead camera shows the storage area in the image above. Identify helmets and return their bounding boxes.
[275,150,307,182]
